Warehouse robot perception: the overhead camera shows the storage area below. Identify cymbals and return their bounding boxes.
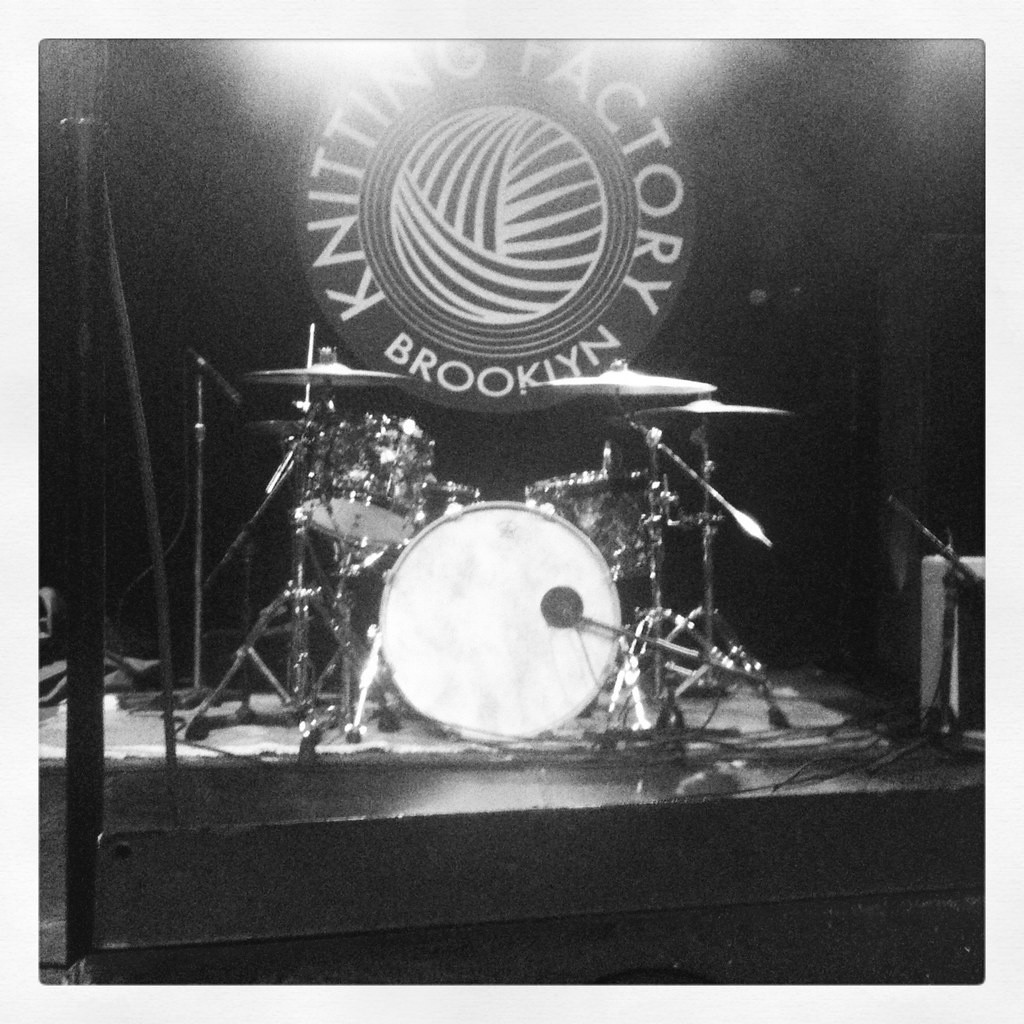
[246,359,415,392]
[527,370,720,400]
[615,400,796,427]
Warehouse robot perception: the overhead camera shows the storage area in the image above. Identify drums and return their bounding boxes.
[286,408,437,553]
[384,474,482,567]
[376,498,626,745]
[522,465,666,585]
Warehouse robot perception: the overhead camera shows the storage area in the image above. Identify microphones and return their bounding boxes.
[183,353,242,405]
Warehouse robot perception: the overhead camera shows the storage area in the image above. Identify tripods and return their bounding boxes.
[865,494,984,776]
[174,415,787,764]
